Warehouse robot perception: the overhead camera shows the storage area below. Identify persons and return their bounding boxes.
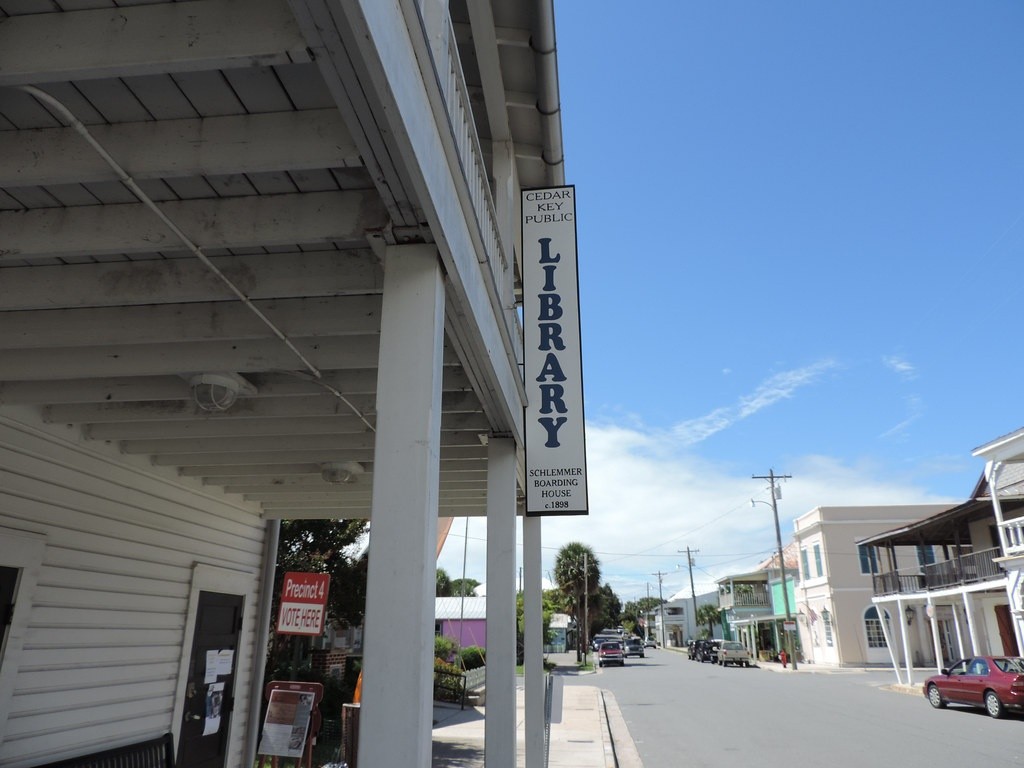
[780,649,786,668]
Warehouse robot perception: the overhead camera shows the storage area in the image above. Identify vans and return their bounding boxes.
[644,636,657,649]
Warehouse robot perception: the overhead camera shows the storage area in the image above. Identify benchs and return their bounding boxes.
[30,733,177,768]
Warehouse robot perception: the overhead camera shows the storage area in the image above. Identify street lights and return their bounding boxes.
[675,545,700,640]
[748,468,797,674]
[649,570,667,648]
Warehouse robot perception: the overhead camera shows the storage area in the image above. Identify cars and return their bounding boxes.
[687,639,749,668]
[591,626,631,651]
[922,656,1024,718]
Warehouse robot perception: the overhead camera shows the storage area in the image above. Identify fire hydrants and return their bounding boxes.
[778,648,788,669]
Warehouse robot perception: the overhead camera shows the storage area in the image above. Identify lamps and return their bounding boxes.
[189,374,241,413]
[797,610,810,628]
[821,607,834,627]
[905,605,915,625]
[320,462,366,485]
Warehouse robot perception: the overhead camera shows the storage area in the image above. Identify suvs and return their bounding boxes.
[625,638,645,658]
[597,642,625,667]
